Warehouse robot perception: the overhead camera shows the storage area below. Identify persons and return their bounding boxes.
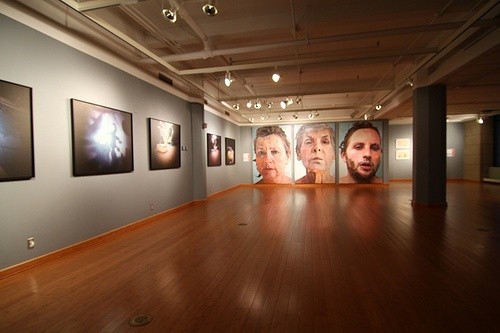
[295,123,336,183]
[339,121,383,183]
[254,125,293,184]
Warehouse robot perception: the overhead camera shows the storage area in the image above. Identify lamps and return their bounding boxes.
[162,0,185,24]
[202,0,219,16]
[233,97,302,110]
[249,112,319,122]
[272,67,282,83]
[224,71,233,87]
